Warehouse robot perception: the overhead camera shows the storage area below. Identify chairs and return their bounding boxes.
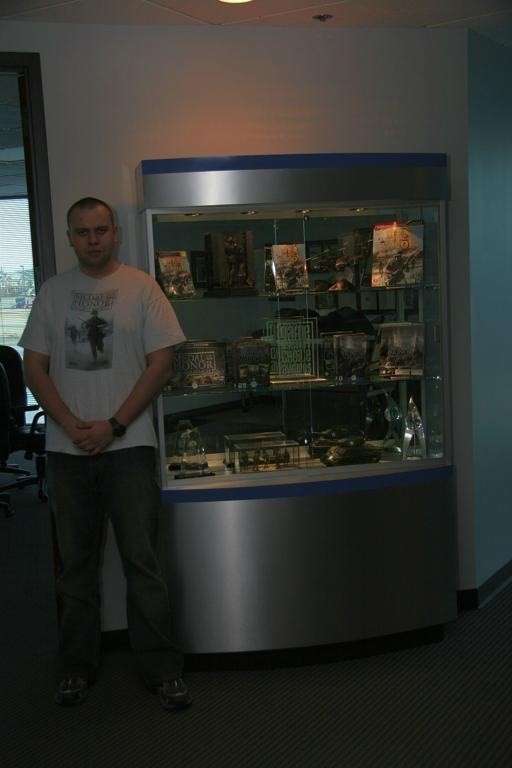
[0,347,48,503]
[0,362,26,518]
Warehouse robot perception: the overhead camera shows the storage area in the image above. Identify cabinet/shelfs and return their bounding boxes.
[133,150,461,669]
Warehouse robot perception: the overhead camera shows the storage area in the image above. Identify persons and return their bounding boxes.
[15,197,196,708]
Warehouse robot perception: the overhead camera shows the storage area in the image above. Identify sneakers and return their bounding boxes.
[52,675,87,714]
[159,675,192,709]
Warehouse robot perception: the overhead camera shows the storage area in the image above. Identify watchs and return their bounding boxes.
[107,417,127,439]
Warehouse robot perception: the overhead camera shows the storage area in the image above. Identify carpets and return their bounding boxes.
[0,480,512,768]
